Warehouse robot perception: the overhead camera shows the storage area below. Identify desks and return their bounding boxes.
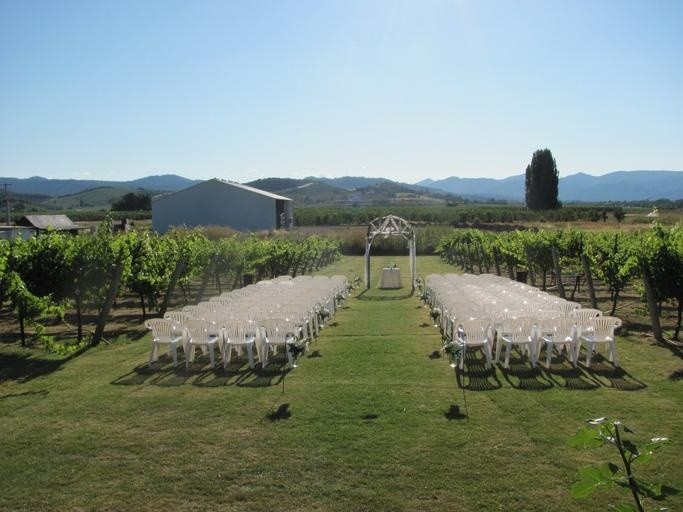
[376,268,402,289]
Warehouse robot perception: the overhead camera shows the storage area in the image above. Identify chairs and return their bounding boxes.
[424,271,624,370]
[144,273,352,371]
